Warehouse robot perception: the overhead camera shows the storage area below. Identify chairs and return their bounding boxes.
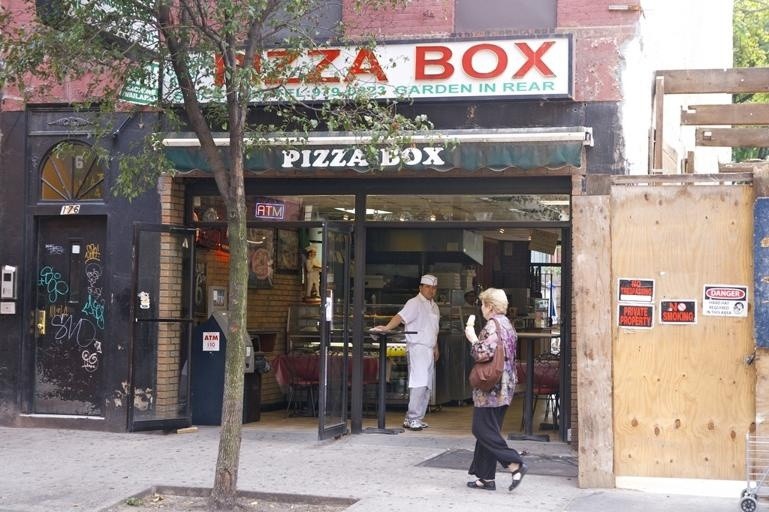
[515,352,560,432]
[282,349,374,418]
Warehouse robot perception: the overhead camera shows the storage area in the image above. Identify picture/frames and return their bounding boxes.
[276,225,301,274]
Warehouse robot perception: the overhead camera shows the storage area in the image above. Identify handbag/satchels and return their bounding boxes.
[468,317,506,394]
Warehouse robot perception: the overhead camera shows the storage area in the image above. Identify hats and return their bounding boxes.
[420,274,438,287]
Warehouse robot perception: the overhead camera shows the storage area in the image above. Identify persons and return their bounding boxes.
[376,275,441,431]
[464,288,528,491]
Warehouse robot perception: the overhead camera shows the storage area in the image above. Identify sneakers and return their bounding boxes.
[403,418,429,430]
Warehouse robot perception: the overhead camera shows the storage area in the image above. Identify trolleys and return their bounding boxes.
[741,432,769,512]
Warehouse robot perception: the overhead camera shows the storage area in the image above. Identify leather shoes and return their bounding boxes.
[467,479,496,490]
[509,462,528,491]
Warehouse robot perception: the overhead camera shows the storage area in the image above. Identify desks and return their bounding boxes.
[278,355,392,416]
[507,333,560,443]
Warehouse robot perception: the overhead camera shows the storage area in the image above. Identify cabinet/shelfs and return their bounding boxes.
[286,301,487,414]
